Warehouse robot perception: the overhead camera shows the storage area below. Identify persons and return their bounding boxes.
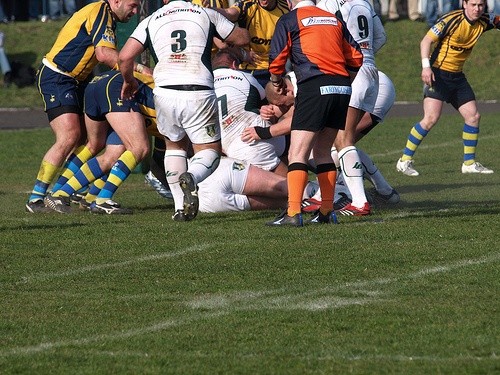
[0,0,500,228]
[396,0,492,175]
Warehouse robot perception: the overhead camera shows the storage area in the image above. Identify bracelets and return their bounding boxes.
[254,126,273,139]
[422,57,431,68]
[135,63,144,73]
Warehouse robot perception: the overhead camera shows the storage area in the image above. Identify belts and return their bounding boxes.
[159,85,214,91]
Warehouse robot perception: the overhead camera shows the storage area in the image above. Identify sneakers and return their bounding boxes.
[301,199,312,213]
[79,196,90,212]
[44,192,74,214]
[302,199,323,213]
[333,192,352,212]
[336,202,371,216]
[307,207,338,224]
[396,158,419,177]
[89,199,134,215]
[264,210,303,227]
[366,187,400,204]
[462,162,494,174]
[25,199,59,214]
[70,192,88,204]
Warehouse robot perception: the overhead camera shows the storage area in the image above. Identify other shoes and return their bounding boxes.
[141,159,173,200]
[4,72,27,88]
[171,210,183,221]
[179,172,199,222]
